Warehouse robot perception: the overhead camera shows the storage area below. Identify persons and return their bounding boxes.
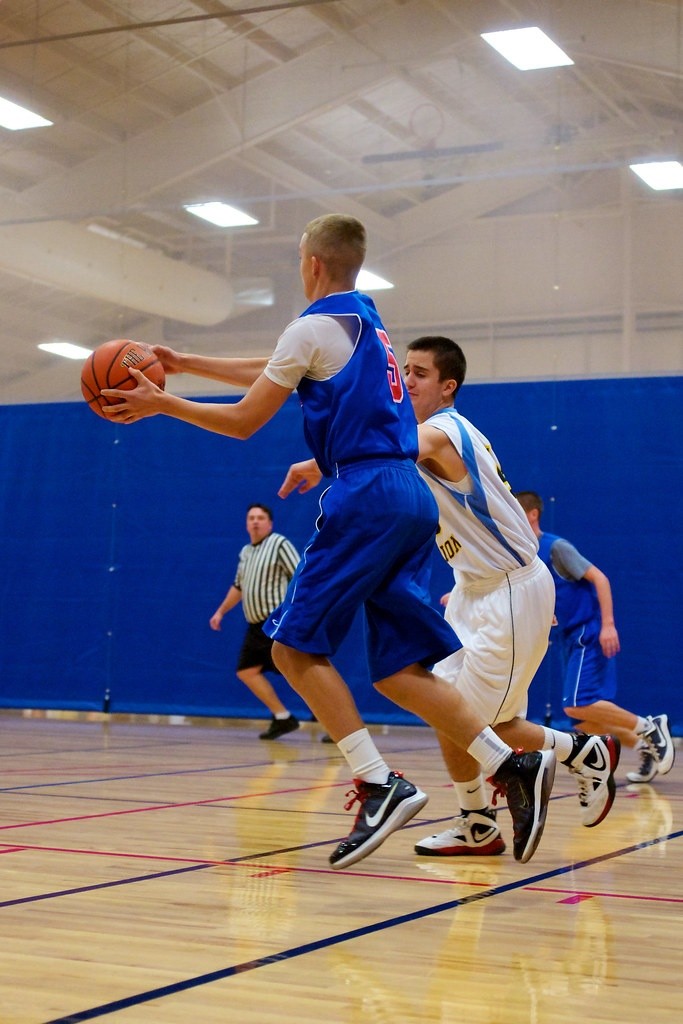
[441,491,674,783]
[100,212,557,869]
[208,504,335,743]
[278,337,622,856]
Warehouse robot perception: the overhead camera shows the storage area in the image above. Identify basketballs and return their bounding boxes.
[84,340,170,422]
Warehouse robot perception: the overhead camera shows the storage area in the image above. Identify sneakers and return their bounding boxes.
[259,713,299,739]
[626,743,658,782]
[414,809,506,856]
[569,732,621,828]
[323,735,334,743]
[646,714,675,775]
[485,745,556,864]
[329,770,429,870]
